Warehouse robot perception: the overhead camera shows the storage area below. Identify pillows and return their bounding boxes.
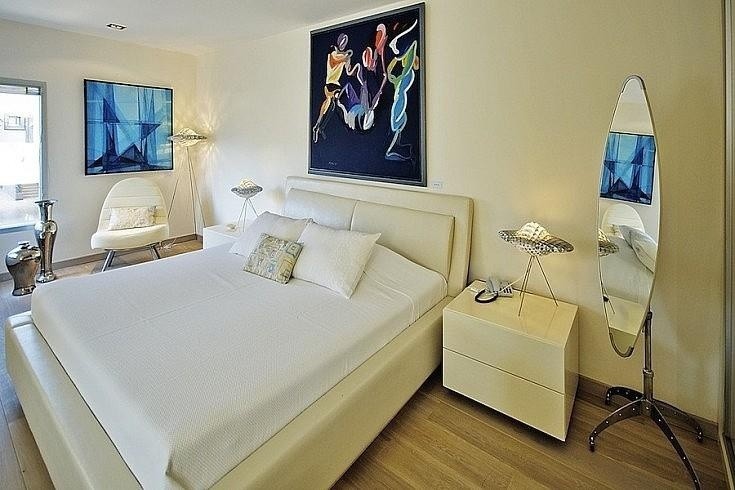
[230,211,381,299]
[107,205,156,230]
[619,226,657,274]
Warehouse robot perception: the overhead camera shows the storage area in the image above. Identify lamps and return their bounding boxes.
[165,128,207,241]
[598,229,620,323]
[499,223,573,316]
[231,179,263,233]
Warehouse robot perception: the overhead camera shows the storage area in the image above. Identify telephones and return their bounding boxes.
[487,277,513,299]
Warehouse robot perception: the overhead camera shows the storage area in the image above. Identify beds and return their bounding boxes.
[7,177,474,489]
[598,234,654,336]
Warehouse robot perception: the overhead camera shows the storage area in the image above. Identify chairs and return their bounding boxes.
[91,177,169,272]
[599,205,645,237]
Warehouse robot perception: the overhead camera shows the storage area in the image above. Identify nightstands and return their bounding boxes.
[441,280,579,443]
[202,219,251,249]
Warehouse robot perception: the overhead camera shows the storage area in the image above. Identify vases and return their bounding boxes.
[5,240,41,297]
[32,199,58,283]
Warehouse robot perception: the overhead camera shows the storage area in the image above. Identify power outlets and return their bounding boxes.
[429,180,444,193]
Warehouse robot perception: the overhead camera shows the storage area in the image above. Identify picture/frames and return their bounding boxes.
[84,78,175,178]
[599,130,657,209]
[308,2,428,188]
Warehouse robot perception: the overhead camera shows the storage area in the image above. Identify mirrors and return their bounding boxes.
[587,76,707,490]
